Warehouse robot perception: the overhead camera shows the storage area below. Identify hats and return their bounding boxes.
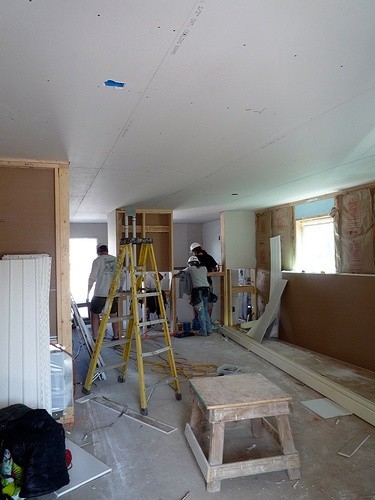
[96,242,107,249]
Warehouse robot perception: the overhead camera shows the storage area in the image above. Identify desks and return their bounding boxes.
[186,372,301,492]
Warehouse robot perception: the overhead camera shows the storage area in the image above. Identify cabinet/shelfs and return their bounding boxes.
[106,208,175,336]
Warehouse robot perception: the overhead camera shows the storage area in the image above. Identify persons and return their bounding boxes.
[189,242,219,284]
[183,256,213,338]
[88,243,124,342]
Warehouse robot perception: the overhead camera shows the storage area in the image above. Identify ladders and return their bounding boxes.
[81,235,182,416]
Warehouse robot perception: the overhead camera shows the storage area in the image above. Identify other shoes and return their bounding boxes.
[207,332,213,336]
[196,332,205,336]
[112,336,119,340]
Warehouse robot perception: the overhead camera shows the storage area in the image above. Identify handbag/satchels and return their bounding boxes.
[0,404,71,498]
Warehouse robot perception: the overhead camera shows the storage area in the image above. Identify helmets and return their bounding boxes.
[189,242,202,254]
[187,255,200,265]
